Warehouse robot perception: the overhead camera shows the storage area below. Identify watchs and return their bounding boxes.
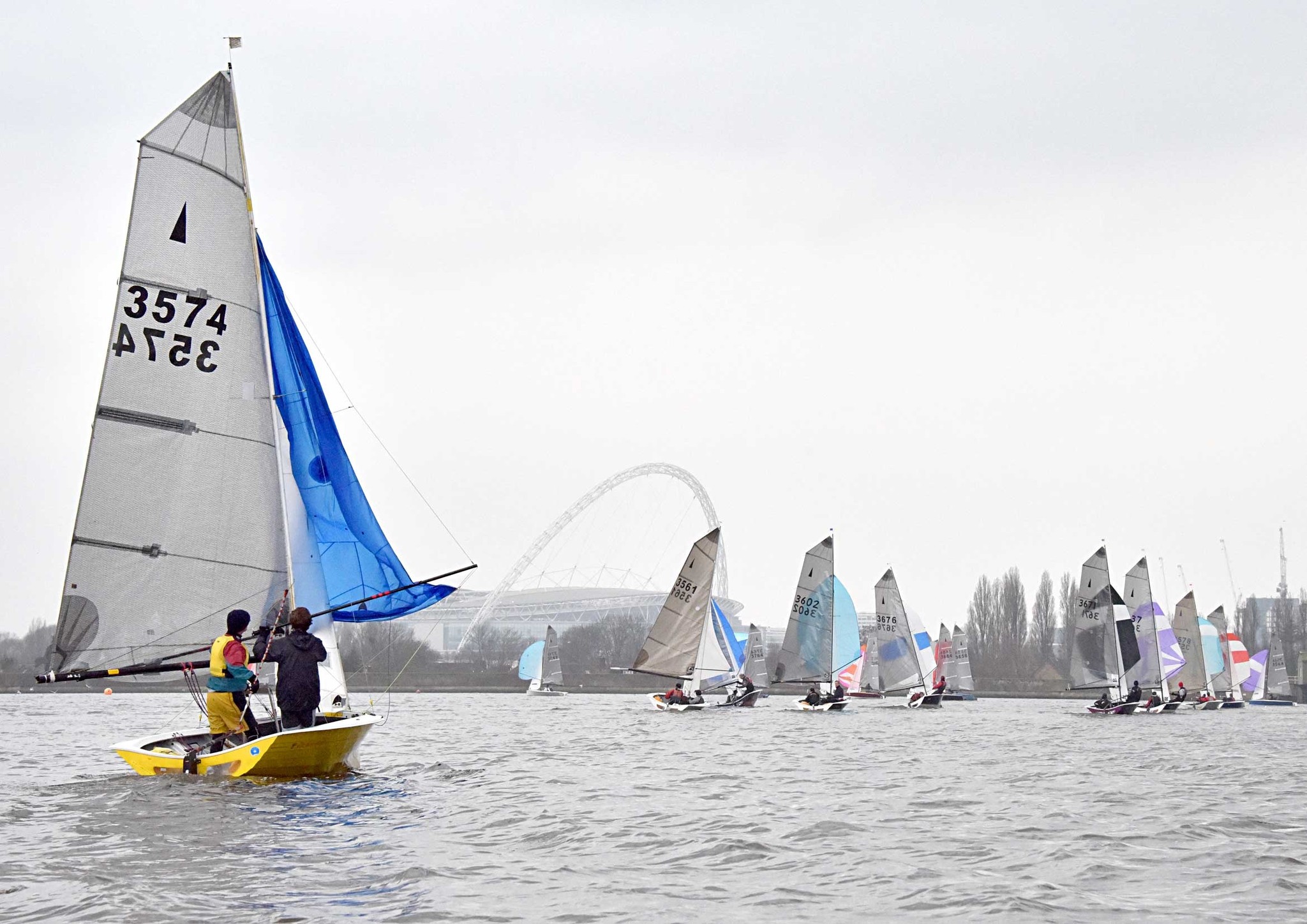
[1206,692,1216,702]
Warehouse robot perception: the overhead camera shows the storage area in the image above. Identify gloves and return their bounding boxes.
[248,674,259,694]
[259,625,271,638]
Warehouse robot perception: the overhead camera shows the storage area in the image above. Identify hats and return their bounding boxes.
[739,674,744,676]
[1178,682,1183,687]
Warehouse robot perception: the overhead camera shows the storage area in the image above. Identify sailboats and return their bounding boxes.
[874,566,944,709]
[933,622,977,701]
[1065,545,1140,715]
[519,624,568,697]
[1124,556,1187,714]
[1242,640,1294,706]
[726,623,769,707]
[628,525,745,712]
[34,37,476,779]
[839,636,885,699]
[771,528,863,712]
[1167,589,1225,710]
[1207,604,1251,708]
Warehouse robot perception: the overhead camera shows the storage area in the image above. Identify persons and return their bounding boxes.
[735,674,754,695]
[1144,692,1161,708]
[1197,694,1206,704]
[931,676,946,694]
[910,691,924,703]
[806,688,819,706]
[1169,682,1186,703]
[1226,694,1234,702]
[666,683,684,702]
[206,609,260,753]
[1126,681,1142,704]
[1097,693,1113,709]
[831,681,843,698]
[865,683,872,693]
[688,690,704,704]
[727,690,738,703]
[252,607,327,732]
